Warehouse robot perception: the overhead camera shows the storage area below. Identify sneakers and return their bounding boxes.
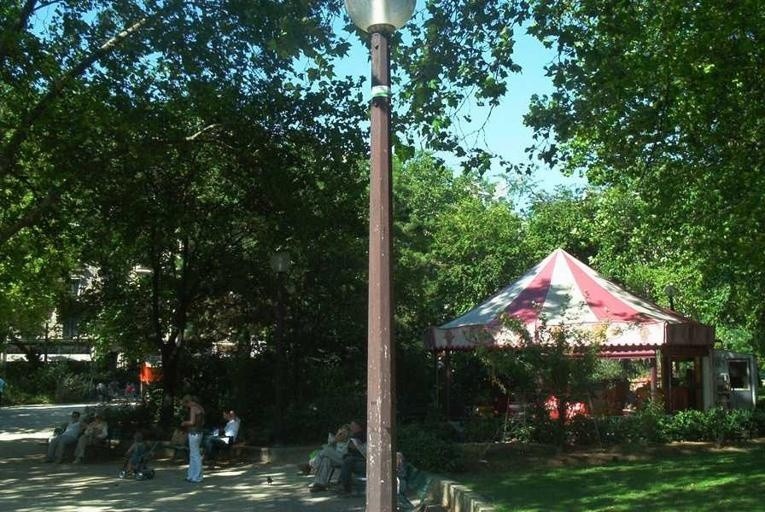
[309,484,326,493]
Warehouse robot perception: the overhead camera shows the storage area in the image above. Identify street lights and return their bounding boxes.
[338,0,415,509]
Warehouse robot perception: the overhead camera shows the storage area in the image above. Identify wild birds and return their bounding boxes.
[266,476,274,484]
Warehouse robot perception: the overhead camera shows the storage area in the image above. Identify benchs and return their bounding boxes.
[160,434,247,470]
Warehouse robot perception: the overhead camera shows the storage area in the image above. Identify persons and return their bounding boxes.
[292,414,367,499]
[179,393,240,485]
[124,432,147,480]
[95,379,137,403]
[38,411,87,464]
[71,411,109,465]
